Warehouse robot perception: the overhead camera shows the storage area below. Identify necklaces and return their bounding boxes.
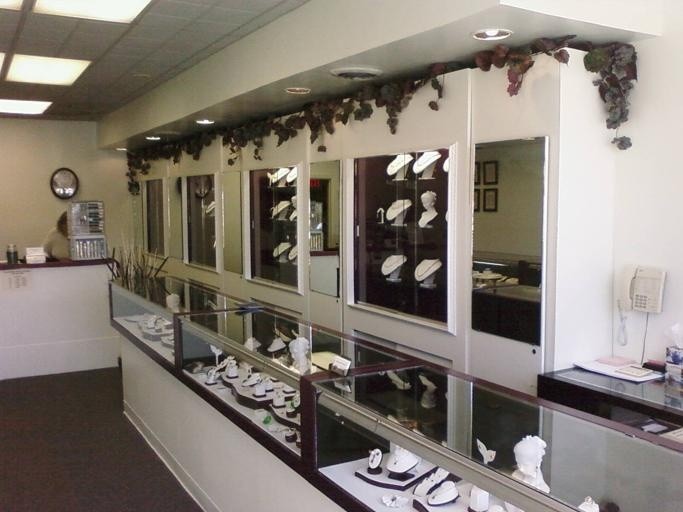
[136,152,547,512]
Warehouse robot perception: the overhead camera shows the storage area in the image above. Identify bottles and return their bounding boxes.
[6,244,17,265]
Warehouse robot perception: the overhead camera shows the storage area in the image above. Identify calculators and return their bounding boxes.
[234,302,264,310]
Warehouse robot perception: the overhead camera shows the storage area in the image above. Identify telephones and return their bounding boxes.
[615,264,666,313]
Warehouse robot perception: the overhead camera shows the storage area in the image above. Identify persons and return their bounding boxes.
[40,210,70,259]
